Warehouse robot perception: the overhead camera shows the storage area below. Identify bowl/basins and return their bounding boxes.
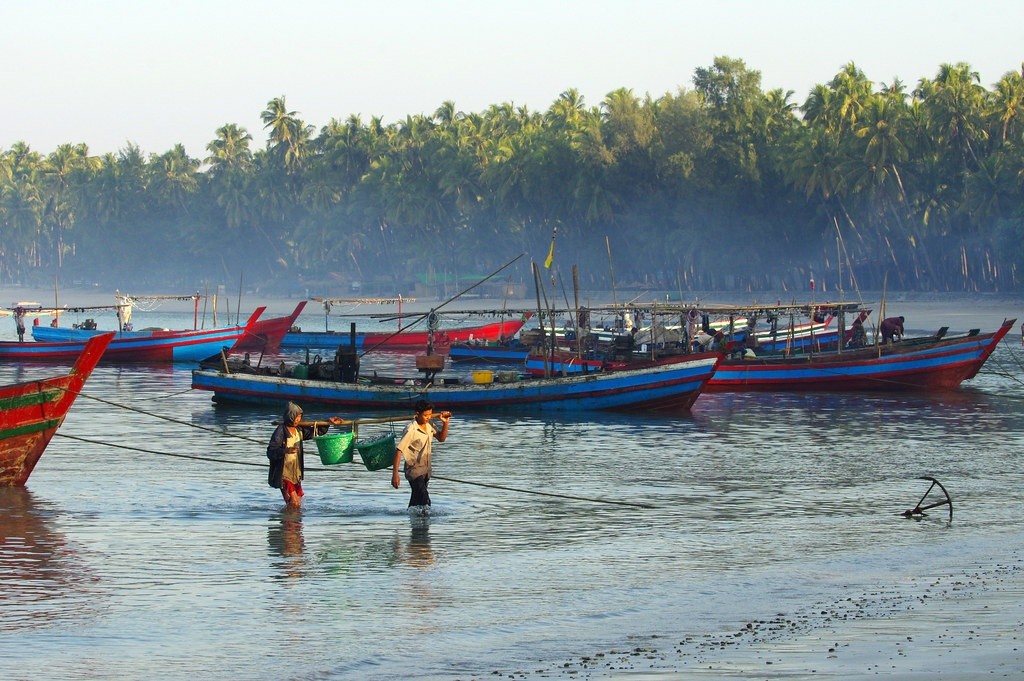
[473,372,494,383]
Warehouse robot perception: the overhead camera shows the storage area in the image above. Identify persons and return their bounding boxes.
[693,329,720,354]
[267,401,343,504]
[615,327,638,349]
[735,345,747,360]
[13,306,26,342]
[391,400,450,509]
[881,316,905,345]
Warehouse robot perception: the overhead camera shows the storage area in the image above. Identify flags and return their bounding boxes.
[544,240,553,271]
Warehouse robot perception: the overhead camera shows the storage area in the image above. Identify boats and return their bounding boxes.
[0,330,117,488]
[279,233,1019,394]
[0,275,251,364]
[189,300,726,415]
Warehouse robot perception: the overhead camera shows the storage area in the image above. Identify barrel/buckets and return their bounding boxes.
[313,431,355,465]
[354,432,396,471]
